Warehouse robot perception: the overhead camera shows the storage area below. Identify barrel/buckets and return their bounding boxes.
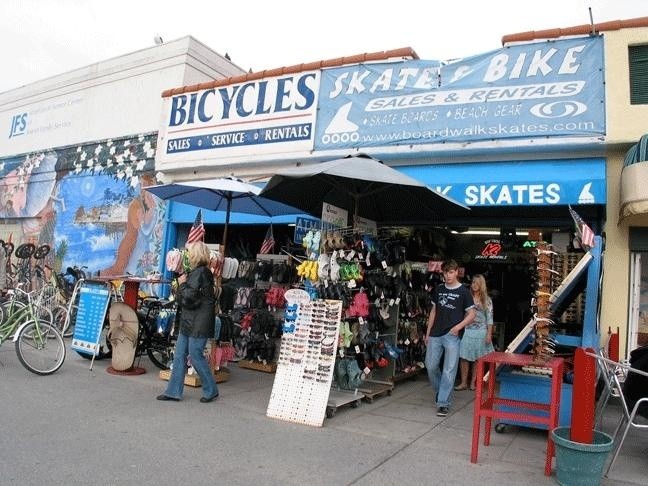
[551,426,614,486]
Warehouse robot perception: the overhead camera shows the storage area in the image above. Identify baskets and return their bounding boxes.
[33,287,59,316]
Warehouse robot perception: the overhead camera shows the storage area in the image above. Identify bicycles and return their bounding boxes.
[0,262,180,377]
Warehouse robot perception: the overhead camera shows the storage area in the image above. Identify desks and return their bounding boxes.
[96,274,173,376]
[471,351,565,476]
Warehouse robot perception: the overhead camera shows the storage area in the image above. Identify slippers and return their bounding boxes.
[107,302,139,371]
[295,231,340,299]
[210,251,291,371]
[339,232,445,390]
[167,250,191,274]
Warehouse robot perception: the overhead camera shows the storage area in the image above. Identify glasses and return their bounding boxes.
[530,248,564,353]
[567,255,578,274]
[566,306,578,323]
[291,300,339,382]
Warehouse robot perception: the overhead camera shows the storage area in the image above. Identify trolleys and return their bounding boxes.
[493,334,581,436]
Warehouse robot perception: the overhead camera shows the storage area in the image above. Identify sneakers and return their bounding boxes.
[200,393,218,402]
[454,383,475,390]
[157,395,180,401]
[435,393,448,416]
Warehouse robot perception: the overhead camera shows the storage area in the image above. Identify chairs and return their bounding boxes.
[585,348,648,478]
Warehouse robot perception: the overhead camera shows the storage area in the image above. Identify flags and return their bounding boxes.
[569,208,595,248]
[259,221,276,254]
[187,208,207,244]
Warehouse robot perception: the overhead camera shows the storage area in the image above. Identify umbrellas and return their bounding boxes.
[255,153,473,240]
[141,171,306,256]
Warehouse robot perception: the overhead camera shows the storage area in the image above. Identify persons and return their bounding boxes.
[156,240,220,403]
[453,273,497,392]
[423,257,476,418]
